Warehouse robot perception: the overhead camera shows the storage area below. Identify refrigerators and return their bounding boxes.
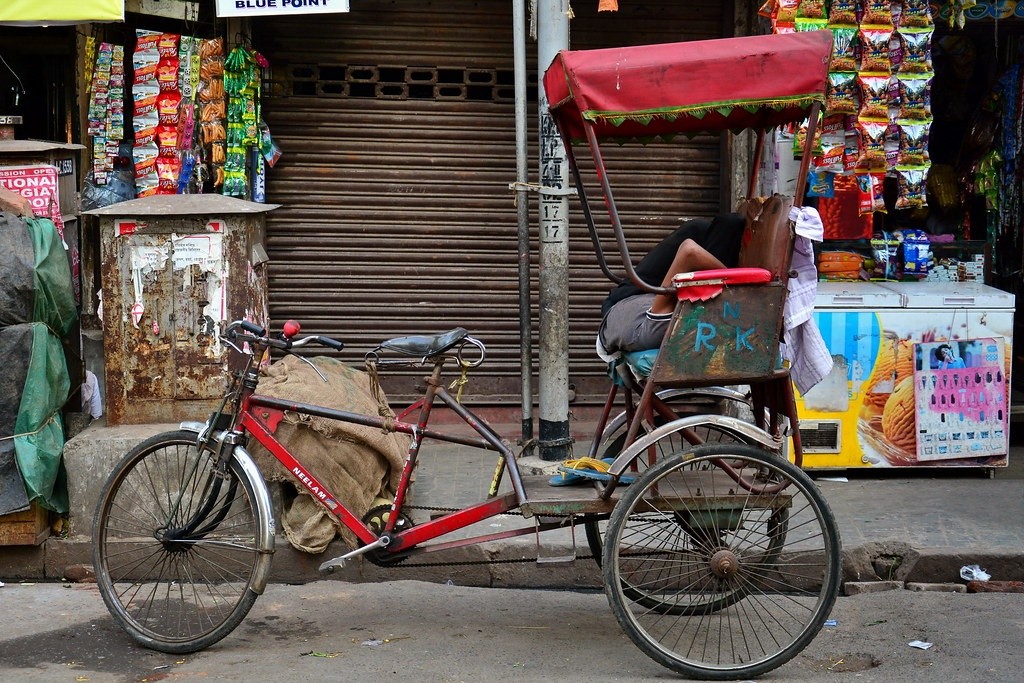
[774,280,1016,481]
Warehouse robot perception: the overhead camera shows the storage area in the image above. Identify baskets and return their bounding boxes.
[856,414,923,467]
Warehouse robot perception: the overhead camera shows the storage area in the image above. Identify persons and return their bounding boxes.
[934,344,966,369]
[599,212,745,354]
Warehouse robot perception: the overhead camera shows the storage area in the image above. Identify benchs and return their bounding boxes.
[623,194,790,382]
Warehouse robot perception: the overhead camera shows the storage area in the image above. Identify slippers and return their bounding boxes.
[559,458,635,483]
[548,455,614,485]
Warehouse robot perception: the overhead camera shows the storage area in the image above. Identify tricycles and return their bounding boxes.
[91,29,843,682]
